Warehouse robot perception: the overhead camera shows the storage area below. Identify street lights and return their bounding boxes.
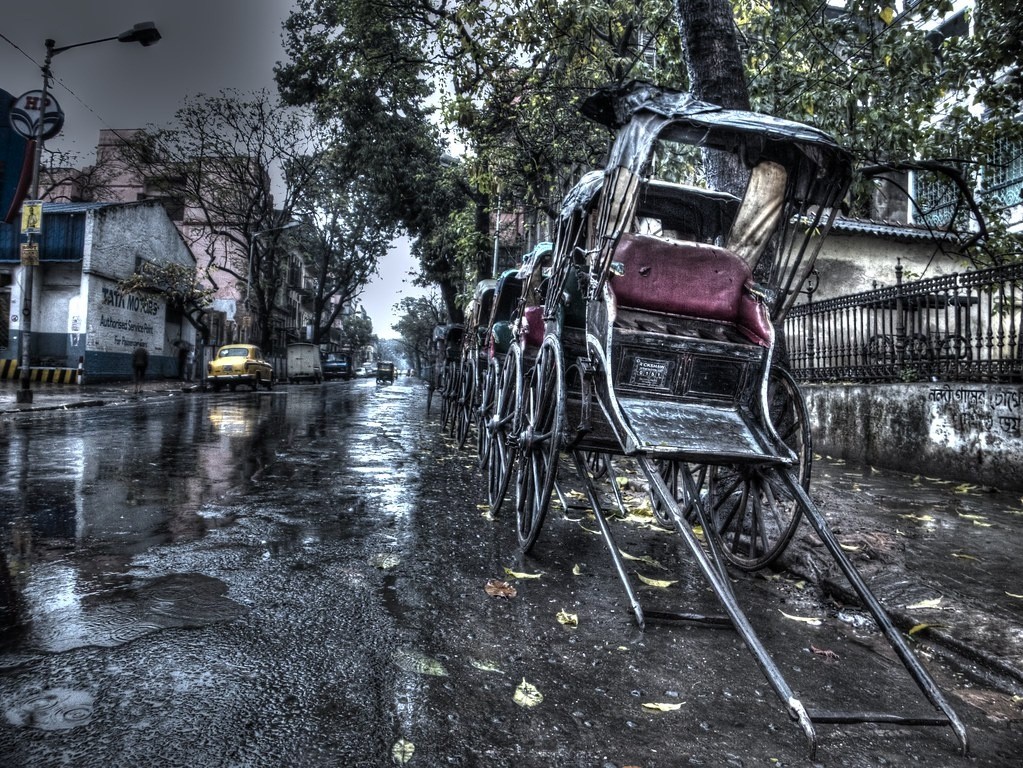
[243,221,300,342]
[12,22,163,396]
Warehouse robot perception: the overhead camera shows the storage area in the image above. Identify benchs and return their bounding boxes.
[520,304,545,378]
[596,232,775,407]
[557,263,589,397]
[477,320,512,365]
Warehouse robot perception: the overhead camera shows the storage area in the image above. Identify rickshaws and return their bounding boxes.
[423,91,971,758]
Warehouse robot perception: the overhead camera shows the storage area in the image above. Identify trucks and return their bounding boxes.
[287,343,324,383]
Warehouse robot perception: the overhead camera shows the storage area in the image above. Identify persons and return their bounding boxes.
[186,351,194,383]
[179,348,187,382]
[131,343,149,393]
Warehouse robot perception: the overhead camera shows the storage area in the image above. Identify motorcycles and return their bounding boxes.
[375,362,394,385]
[411,369,416,376]
[394,367,398,380]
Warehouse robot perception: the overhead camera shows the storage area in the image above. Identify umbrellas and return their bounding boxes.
[173,340,193,351]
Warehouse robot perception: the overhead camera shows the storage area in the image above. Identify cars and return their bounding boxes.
[397,370,401,376]
[356,366,372,378]
[402,370,410,377]
[208,343,277,391]
[324,354,356,380]
